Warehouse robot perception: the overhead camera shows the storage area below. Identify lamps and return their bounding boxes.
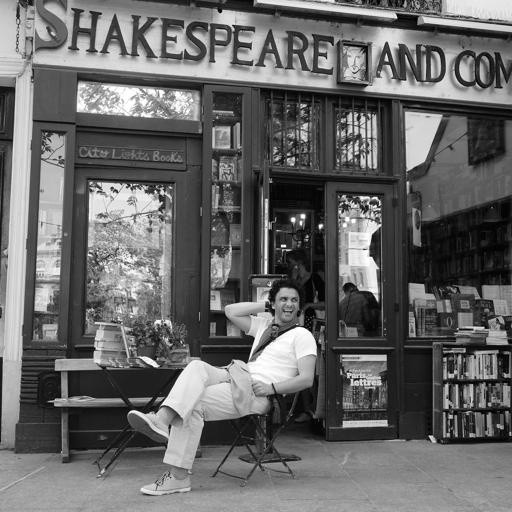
[289,213,324,248]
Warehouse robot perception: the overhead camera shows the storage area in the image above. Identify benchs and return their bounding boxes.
[54,356,202,463]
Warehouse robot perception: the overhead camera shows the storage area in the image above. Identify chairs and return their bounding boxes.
[208,390,301,487]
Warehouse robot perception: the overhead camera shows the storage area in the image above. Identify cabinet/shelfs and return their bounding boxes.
[432,341,512,444]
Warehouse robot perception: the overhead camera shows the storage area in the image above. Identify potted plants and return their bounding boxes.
[133,316,190,367]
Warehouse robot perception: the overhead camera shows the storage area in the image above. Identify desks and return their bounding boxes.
[90,358,187,477]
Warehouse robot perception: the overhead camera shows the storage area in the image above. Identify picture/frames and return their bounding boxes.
[246,273,293,317]
[337,39,373,86]
[467,117,506,165]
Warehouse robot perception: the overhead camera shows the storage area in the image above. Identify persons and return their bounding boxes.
[339,282,382,338]
[126,277,318,498]
[286,249,326,424]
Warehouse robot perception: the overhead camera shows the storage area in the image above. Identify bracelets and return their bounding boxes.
[271,382,278,395]
[265,302,271,312]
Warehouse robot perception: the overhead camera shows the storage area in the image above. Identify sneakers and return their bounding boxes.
[127,410,170,443]
[293,412,312,423]
[140,470,191,496]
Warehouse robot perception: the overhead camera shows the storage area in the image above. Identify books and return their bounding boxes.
[276,222,312,273]
[410,205,512,438]
[209,103,241,336]
